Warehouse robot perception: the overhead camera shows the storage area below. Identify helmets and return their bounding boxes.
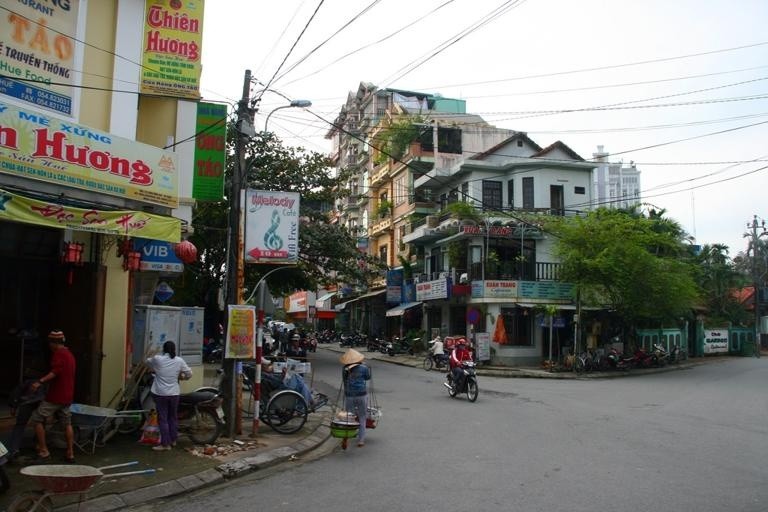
[457,338,467,345]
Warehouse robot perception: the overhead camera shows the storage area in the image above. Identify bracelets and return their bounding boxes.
[38,380,44,384]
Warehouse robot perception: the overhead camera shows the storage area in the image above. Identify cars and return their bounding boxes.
[267,318,296,337]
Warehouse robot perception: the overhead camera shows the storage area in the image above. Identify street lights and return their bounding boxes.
[263,99,315,154]
[744,214,766,359]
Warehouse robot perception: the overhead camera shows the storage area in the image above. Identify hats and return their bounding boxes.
[47,330,64,338]
[339,348,364,364]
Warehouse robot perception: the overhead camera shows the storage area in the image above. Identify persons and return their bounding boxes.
[144,341,193,451]
[451,338,474,390]
[338,348,371,449]
[429,336,444,368]
[29,329,75,464]
[273,324,318,362]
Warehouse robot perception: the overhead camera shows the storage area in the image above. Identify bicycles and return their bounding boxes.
[567,340,682,372]
[421,349,450,372]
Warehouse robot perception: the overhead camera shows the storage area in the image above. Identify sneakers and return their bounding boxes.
[152,441,176,451]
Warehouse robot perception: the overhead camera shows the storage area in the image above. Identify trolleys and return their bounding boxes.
[56,402,151,456]
[5,459,160,512]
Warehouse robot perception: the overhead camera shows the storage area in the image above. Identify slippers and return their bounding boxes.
[62,453,76,463]
[30,454,49,462]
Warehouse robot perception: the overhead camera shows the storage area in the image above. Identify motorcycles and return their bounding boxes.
[113,366,229,446]
[301,327,416,357]
[442,360,479,403]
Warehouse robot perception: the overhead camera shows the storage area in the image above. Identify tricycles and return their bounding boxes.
[190,359,328,435]
